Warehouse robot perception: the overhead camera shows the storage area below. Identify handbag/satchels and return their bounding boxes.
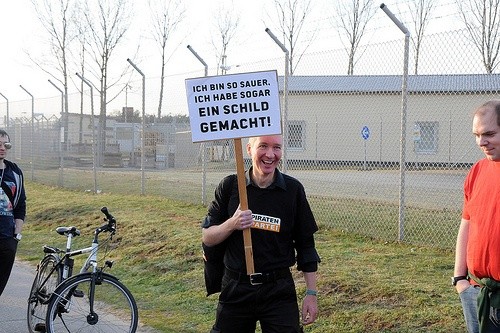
[202,175,234,297]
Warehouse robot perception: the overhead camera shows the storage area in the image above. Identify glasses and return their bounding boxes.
[0,142,12,149]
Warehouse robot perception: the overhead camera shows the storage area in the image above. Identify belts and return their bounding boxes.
[225,266,290,285]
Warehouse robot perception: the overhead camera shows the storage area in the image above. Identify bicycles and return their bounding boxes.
[26,206,139,333]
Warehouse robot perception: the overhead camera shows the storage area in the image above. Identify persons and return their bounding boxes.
[451,101,500,333]
[0,130,26,296]
[202,135,321,333]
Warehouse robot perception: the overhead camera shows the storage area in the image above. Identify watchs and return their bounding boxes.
[13,233,22,241]
[452,276,466,285]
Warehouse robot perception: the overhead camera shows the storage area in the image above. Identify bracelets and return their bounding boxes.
[307,290,317,296]
